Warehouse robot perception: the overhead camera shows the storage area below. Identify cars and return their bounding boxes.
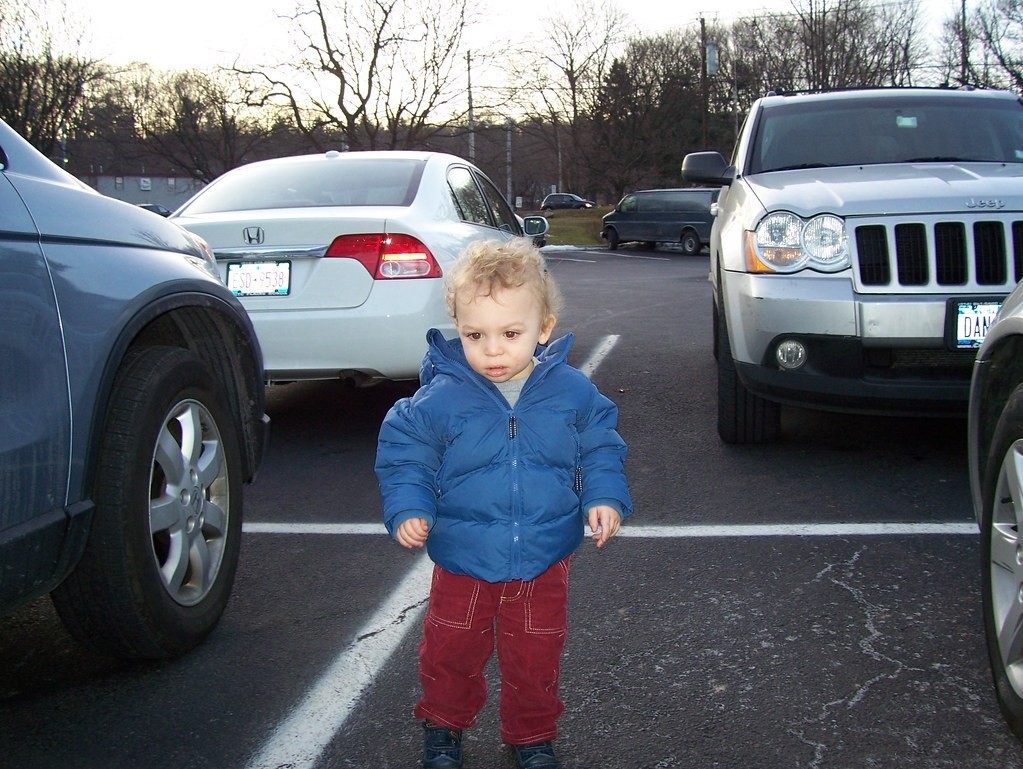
[540,192,596,211]
[164,147,550,408]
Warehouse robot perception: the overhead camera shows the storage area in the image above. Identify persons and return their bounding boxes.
[621,186,636,211]
[373,237,634,769]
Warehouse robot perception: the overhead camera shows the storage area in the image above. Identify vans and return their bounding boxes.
[599,187,723,256]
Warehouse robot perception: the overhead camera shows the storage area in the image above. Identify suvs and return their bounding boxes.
[0,116,273,668]
[679,82,1023,448]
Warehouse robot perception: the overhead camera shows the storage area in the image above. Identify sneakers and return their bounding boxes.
[510,740,559,769]
[421,718,461,769]
